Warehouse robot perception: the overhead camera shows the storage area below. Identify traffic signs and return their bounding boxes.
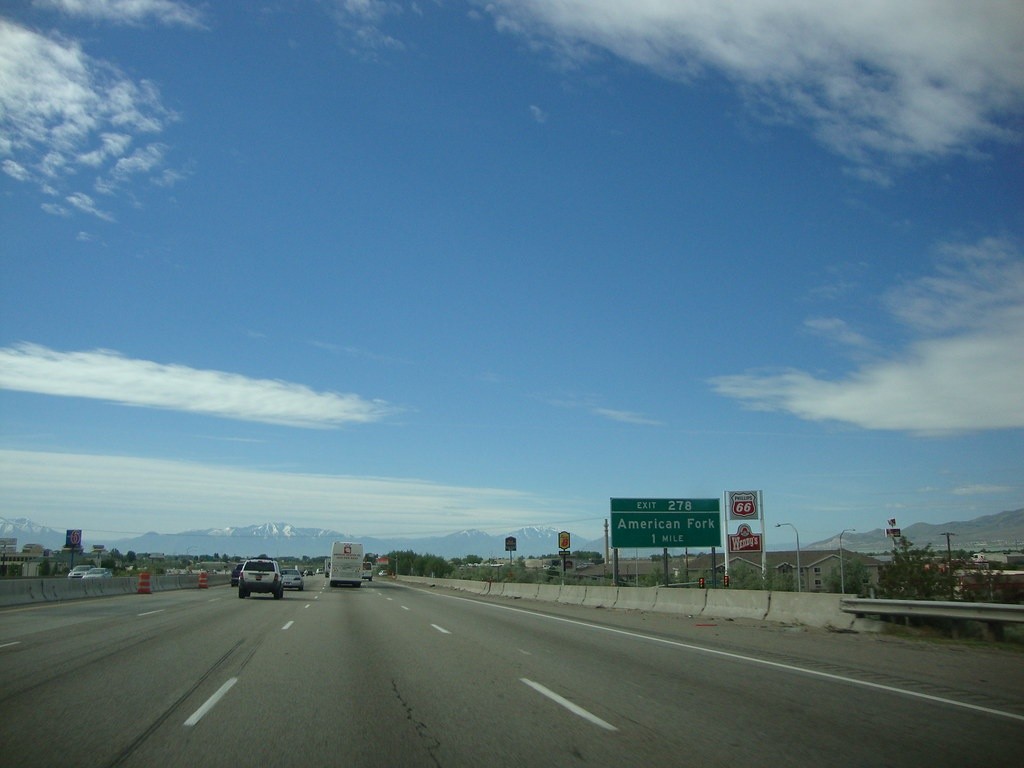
[610,496,723,551]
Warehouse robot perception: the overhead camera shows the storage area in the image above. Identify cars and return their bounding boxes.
[378,570,388,577]
[68,564,96,579]
[280,568,306,591]
[80,567,113,580]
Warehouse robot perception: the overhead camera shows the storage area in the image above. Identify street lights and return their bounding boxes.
[775,523,802,592]
[837,528,857,594]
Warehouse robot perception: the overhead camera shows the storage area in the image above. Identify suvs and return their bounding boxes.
[231,563,245,586]
[238,558,284,599]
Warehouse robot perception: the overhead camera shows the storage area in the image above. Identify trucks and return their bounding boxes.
[324,558,332,578]
[330,541,363,588]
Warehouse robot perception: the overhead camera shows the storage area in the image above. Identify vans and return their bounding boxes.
[362,562,374,581]
